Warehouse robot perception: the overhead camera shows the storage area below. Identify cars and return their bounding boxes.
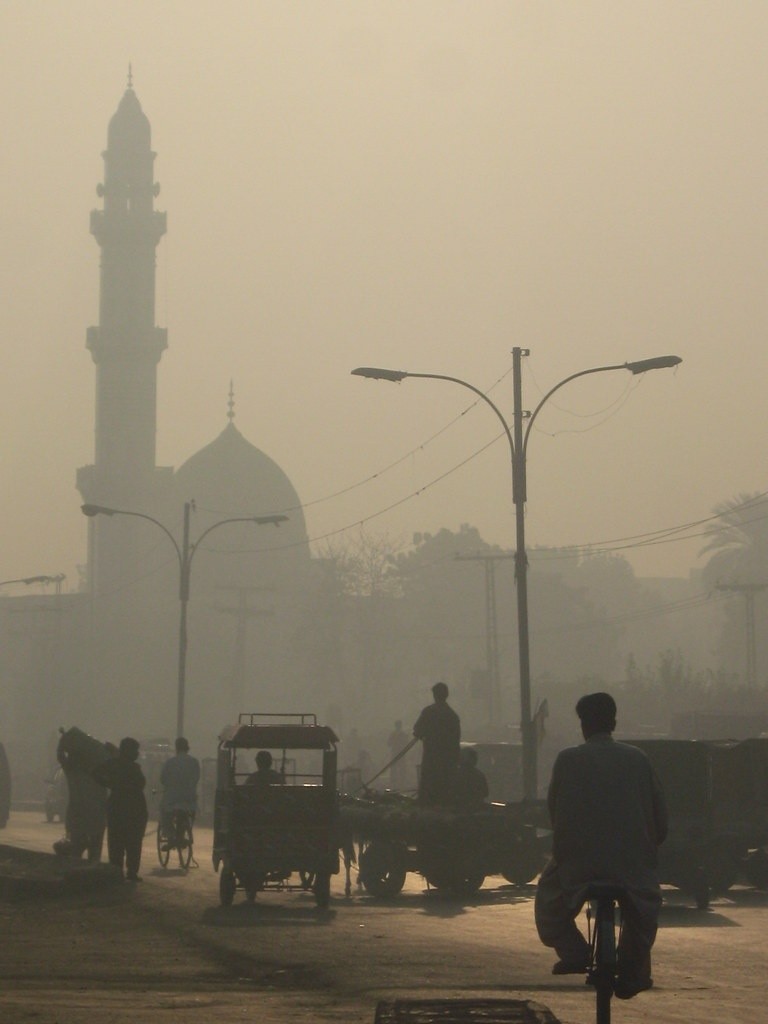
[44,766,70,825]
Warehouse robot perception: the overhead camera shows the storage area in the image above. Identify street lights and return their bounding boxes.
[78,500,293,739]
[349,355,691,798]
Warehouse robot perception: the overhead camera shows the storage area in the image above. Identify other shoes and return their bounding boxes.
[615,976,654,1000]
[552,960,592,975]
[126,873,144,882]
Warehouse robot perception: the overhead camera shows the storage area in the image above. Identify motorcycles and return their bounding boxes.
[211,713,342,906]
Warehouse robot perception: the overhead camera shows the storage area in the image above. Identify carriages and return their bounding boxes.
[298,783,551,900]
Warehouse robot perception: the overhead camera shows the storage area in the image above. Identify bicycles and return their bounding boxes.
[572,882,635,1024]
[151,786,196,870]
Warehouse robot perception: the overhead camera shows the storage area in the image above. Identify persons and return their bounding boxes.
[534,694,667,1000]
[159,737,200,851]
[413,682,460,801]
[388,720,409,782]
[244,751,287,783]
[102,737,148,882]
[53,733,109,861]
[461,748,489,798]
[344,730,360,769]
